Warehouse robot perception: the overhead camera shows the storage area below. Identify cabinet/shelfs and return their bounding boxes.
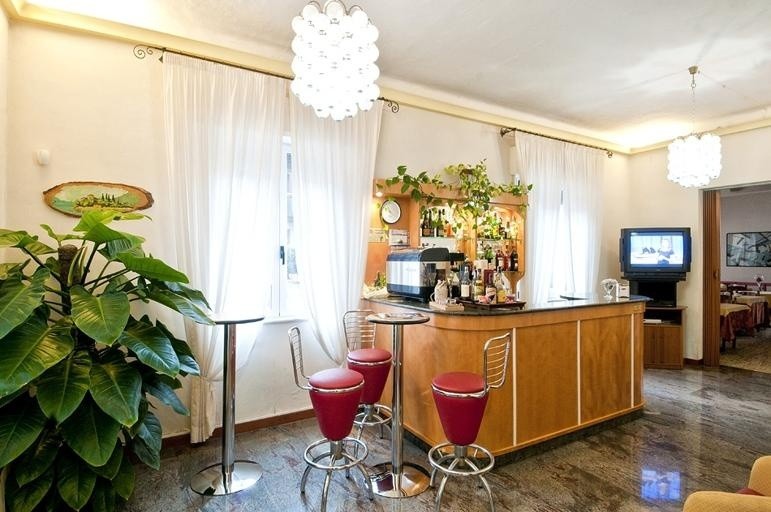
[361,179,528,296]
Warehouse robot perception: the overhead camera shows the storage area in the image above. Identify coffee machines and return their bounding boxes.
[387,245,465,299]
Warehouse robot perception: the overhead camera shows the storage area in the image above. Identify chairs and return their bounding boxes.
[428,332,510,512]
[287,326,374,512]
[683,456,771,512]
[342,310,393,459]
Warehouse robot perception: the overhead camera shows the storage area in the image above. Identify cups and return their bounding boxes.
[601,276,631,300]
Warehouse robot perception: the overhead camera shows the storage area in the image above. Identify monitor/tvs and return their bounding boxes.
[619,226,692,278]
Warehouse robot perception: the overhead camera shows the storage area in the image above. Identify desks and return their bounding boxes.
[720,282,771,356]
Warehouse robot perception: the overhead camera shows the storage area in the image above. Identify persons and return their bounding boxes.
[643,471,673,496]
[643,239,675,264]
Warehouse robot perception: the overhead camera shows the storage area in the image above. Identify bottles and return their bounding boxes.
[510,172,521,191]
[431,265,515,307]
[421,208,455,237]
[476,208,519,271]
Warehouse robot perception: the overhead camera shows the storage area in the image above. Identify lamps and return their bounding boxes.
[667,66,722,187]
[291,0,380,122]
